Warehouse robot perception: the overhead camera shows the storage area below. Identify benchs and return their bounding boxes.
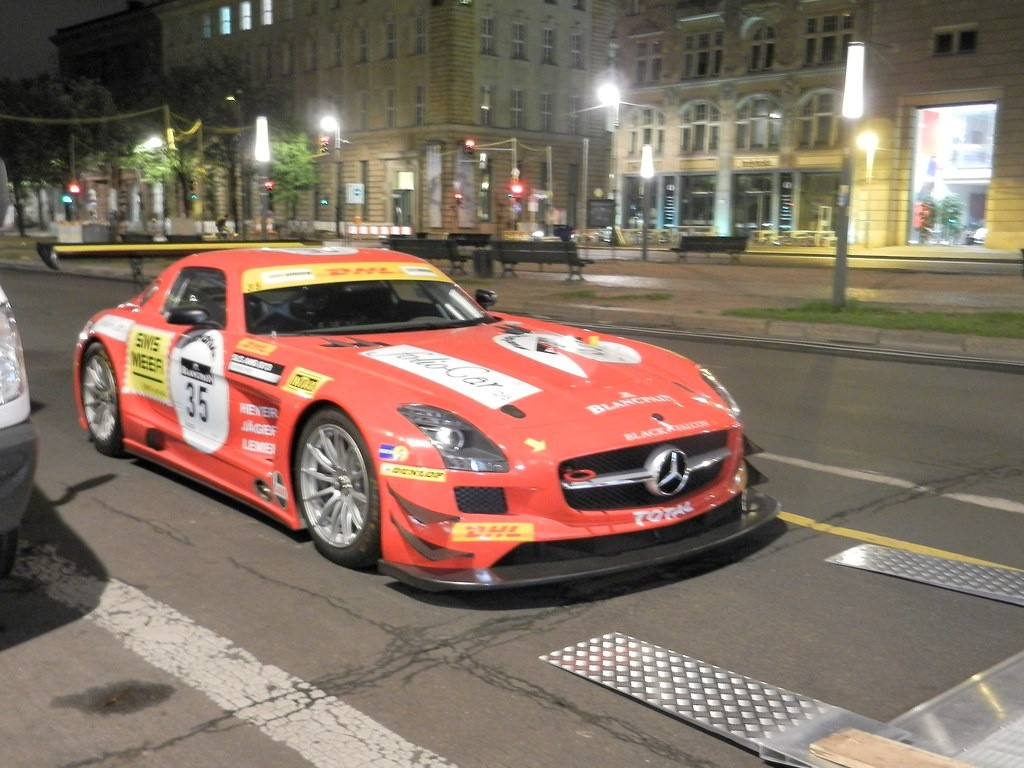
[491,240,594,283]
[670,236,748,265]
[447,233,492,251]
[388,239,473,276]
[752,236,838,248]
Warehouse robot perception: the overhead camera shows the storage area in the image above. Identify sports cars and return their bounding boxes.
[74,245,783,594]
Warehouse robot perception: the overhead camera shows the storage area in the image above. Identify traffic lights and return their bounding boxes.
[465,139,478,153]
[68,182,81,194]
[63,194,74,207]
[511,184,524,198]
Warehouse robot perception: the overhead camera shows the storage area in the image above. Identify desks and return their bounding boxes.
[750,230,778,242]
[808,231,836,247]
[782,230,808,239]
[621,229,641,244]
[648,229,669,246]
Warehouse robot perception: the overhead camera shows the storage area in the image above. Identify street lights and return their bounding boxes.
[321,116,345,238]
